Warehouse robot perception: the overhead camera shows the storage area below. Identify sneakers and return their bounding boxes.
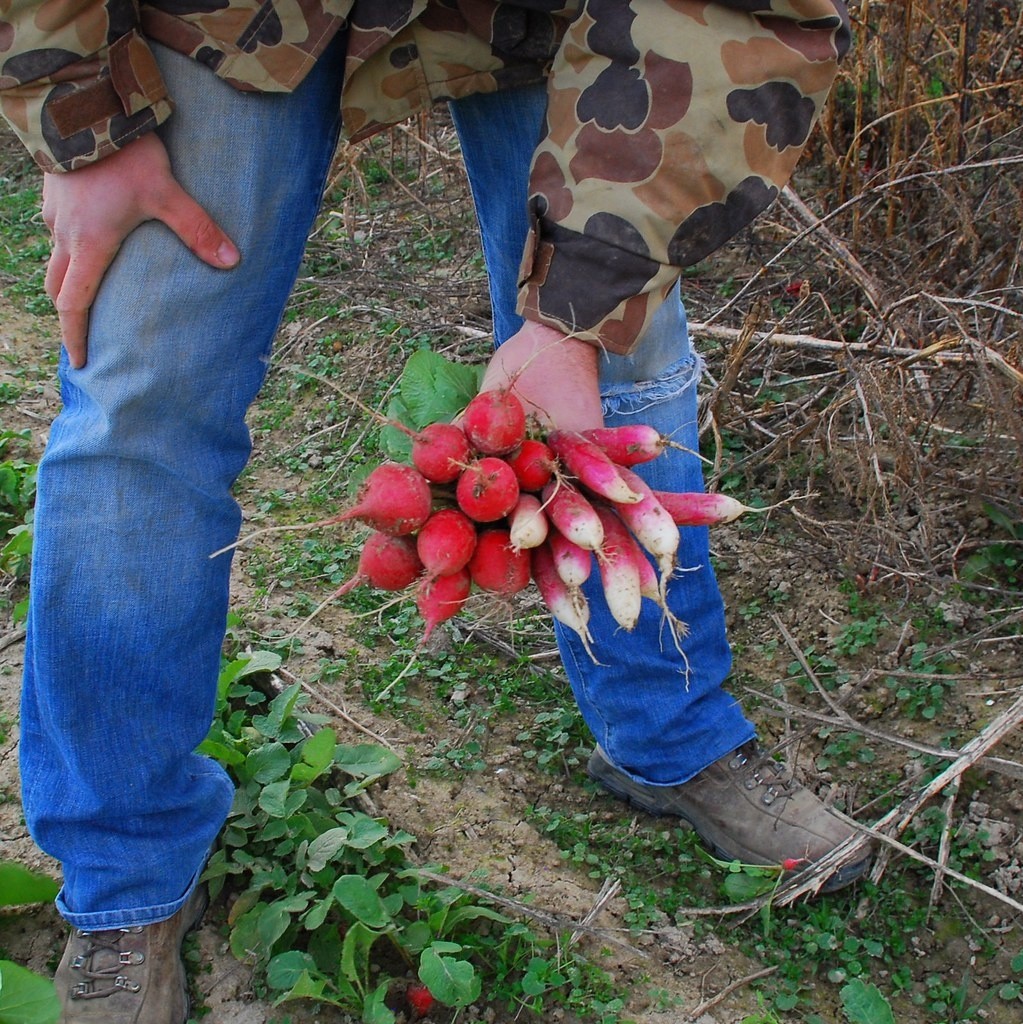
[585,747,872,892]
[51,836,219,1024]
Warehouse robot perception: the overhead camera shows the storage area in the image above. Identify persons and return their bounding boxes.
[0,0,870,1022]
